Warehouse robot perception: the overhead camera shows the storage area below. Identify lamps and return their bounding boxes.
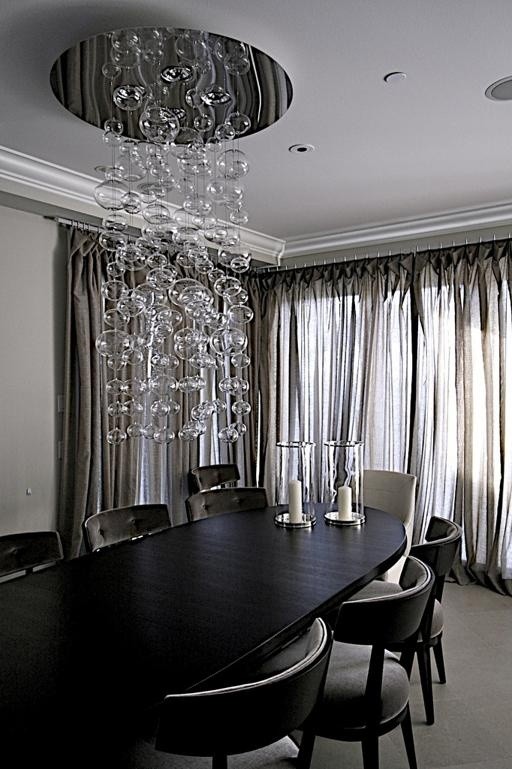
[337,486,352,522]
[287,480,303,523]
[50,26,293,444]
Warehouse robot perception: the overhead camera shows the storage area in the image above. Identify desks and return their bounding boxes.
[1,502,408,768]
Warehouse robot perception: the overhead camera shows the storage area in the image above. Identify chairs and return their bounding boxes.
[329,516,460,725]
[188,465,241,492]
[81,503,172,555]
[362,469,418,588]
[122,616,334,768]
[184,487,269,522]
[2,531,63,582]
[293,556,436,767]
